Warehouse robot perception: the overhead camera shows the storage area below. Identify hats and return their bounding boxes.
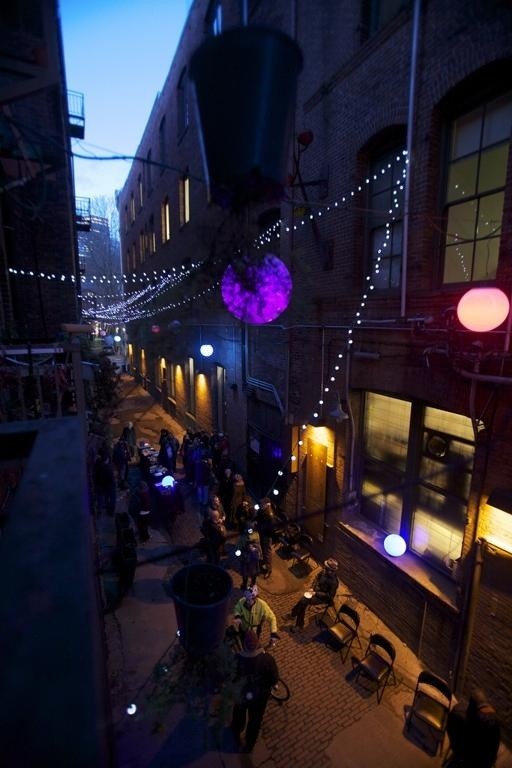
[245,584,257,599]
[325,558,339,571]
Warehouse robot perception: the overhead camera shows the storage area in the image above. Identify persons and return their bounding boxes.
[128,501,152,544]
[179,427,281,590]
[138,453,152,493]
[86,421,137,517]
[156,430,179,473]
[109,512,138,601]
[227,584,280,752]
[281,559,339,632]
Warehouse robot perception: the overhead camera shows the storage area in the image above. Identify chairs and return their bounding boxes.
[308,574,341,626]
[272,512,314,572]
[327,603,363,663]
[402,667,452,757]
[355,633,397,704]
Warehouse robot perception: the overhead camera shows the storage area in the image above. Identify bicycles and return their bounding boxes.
[230,625,290,701]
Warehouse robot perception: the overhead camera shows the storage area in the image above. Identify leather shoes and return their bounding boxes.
[264,569,272,579]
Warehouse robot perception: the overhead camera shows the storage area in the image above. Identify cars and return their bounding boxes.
[103,345,114,355]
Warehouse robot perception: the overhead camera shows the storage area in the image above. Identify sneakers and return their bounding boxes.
[281,611,305,634]
